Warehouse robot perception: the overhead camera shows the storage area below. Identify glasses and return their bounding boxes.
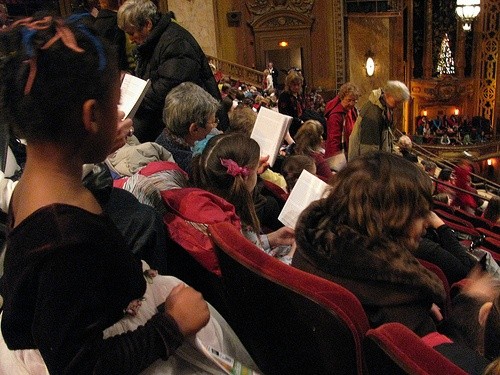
[202,118,220,127]
[344,98,358,103]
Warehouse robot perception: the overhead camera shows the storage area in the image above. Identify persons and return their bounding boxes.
[323,83,360,158]
[210,62,278,112]
[156,81,221,181]
[118,0,220,144]
[281,120,335,191]
[304,83,323,119]
[283,154,317,195]
[0,16,210,375]
[414,210,478,285]
[215,96,234,133]
[291,149,446,336]
[284,67,303,99]
[193,132,297,268]
[278,72,304,119]
[347,81,411,161]
[421,154,500,226]
[226,104,287,172]
[416,110,493,148]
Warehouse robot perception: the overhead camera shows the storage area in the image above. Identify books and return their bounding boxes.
[118,74,151,121]
[251,107,293,167]
[278,169,333,231]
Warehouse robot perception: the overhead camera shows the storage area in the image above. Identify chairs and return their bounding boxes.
[160,174,500,375]
